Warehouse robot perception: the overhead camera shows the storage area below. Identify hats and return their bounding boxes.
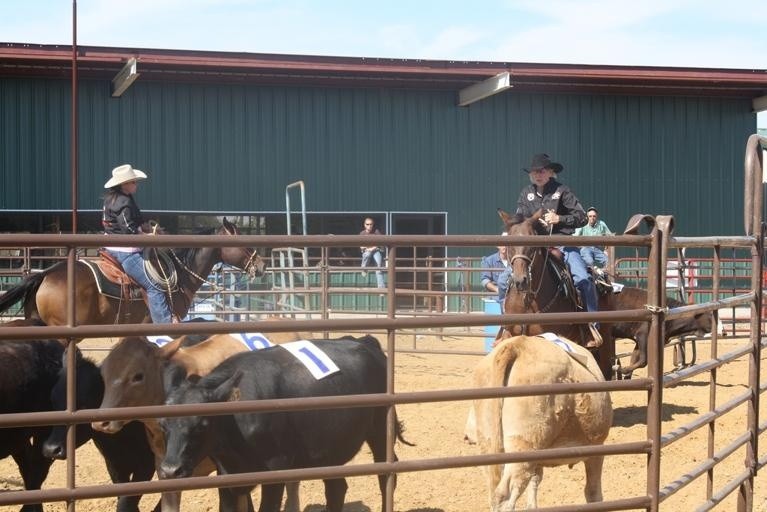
[522,152,564,174]
[103,163,148,189]
[587,206,599,213]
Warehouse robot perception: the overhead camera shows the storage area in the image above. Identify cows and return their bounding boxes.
[611,283,726,380]
[462,332,612,511]
[42,343,162,512]
[90,316,313,512]
[155,335,415,512]
[0,319,63,512]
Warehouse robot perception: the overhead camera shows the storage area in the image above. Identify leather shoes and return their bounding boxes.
[585,328,596,348]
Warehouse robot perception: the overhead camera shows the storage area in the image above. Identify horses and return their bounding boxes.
[0,217,265,348]
[494,205,615,381]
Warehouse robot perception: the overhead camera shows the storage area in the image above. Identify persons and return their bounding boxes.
[572,206,614,276]
[479,230,513,296]
[358,217,389,295]
[101,164,179,326]
[497,154,604,347]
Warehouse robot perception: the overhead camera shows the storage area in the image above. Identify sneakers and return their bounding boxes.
[361,270,367,277]
